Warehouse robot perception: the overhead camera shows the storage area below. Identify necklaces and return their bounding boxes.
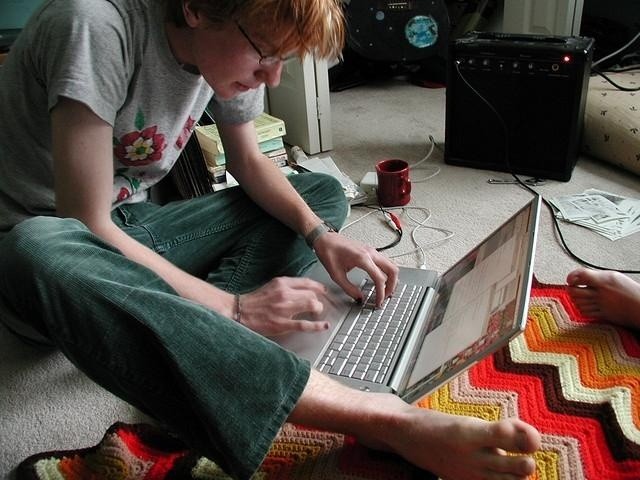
[165,31,186,69]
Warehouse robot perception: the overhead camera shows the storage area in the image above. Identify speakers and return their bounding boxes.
[443,31,597,182]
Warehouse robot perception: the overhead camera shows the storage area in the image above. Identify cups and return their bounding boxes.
[374,159,411,207]
[290,146,308,165]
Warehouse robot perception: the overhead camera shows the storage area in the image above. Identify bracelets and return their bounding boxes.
[232,291,243,324]
[304,221,337,251]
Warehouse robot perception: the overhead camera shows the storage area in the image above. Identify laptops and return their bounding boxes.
[261,191,544,407]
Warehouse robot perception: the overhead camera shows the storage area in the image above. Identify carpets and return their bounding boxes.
[16,284,640,478]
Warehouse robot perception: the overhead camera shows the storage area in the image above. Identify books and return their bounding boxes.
[168,102,369,207]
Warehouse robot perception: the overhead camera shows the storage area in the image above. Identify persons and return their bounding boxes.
[0,0,542,480]
[565,268,640,330]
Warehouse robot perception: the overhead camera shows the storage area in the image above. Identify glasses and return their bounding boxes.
[227,9,301,66]
